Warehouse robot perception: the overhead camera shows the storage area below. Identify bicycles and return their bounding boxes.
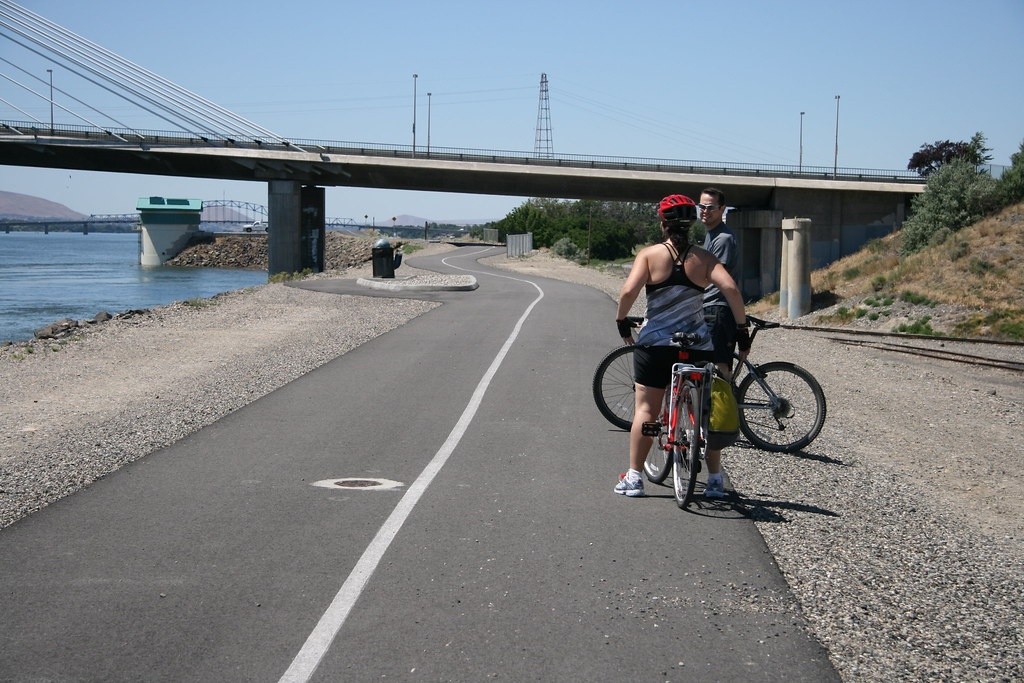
[592,314,828,510]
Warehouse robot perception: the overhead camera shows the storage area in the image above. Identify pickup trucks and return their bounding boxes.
[243,221,268,232]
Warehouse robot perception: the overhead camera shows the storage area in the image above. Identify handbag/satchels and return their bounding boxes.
[706,376,739,450]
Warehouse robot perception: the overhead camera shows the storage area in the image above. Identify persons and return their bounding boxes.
[698,188,741,442]
[616,194,751,497]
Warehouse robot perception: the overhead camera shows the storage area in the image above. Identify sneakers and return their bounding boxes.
[614,472,645,496]
[703,479,724,497]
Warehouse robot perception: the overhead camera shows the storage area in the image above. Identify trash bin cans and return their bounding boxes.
[372,239,395,278]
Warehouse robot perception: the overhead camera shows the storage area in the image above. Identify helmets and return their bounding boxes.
[659,194,697,221]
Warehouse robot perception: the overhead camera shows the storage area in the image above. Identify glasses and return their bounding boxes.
[699,203,720,209]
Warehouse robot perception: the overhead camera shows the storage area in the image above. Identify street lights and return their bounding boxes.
[47,69,54,135]
[833,93,840,179]
[412,73,418,159]
[799,111,805,175]
[427,92,432,158]
